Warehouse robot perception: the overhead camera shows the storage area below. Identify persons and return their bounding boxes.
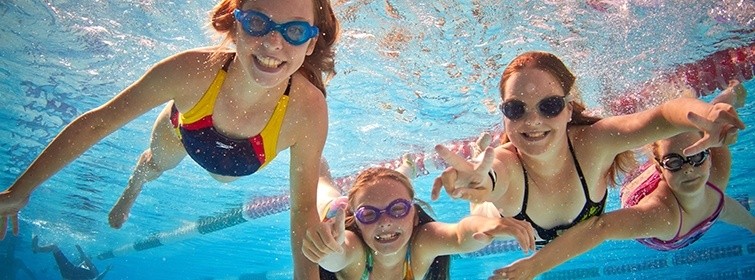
[490,82,755,280]
[302,157,537,280]
[431,52,745,250]
[0,0,340,280]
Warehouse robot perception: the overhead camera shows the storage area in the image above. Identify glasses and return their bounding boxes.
[233,8,319,45]
[654,150,709,173]
[356,199,411,224]
[499,96,567,121]
